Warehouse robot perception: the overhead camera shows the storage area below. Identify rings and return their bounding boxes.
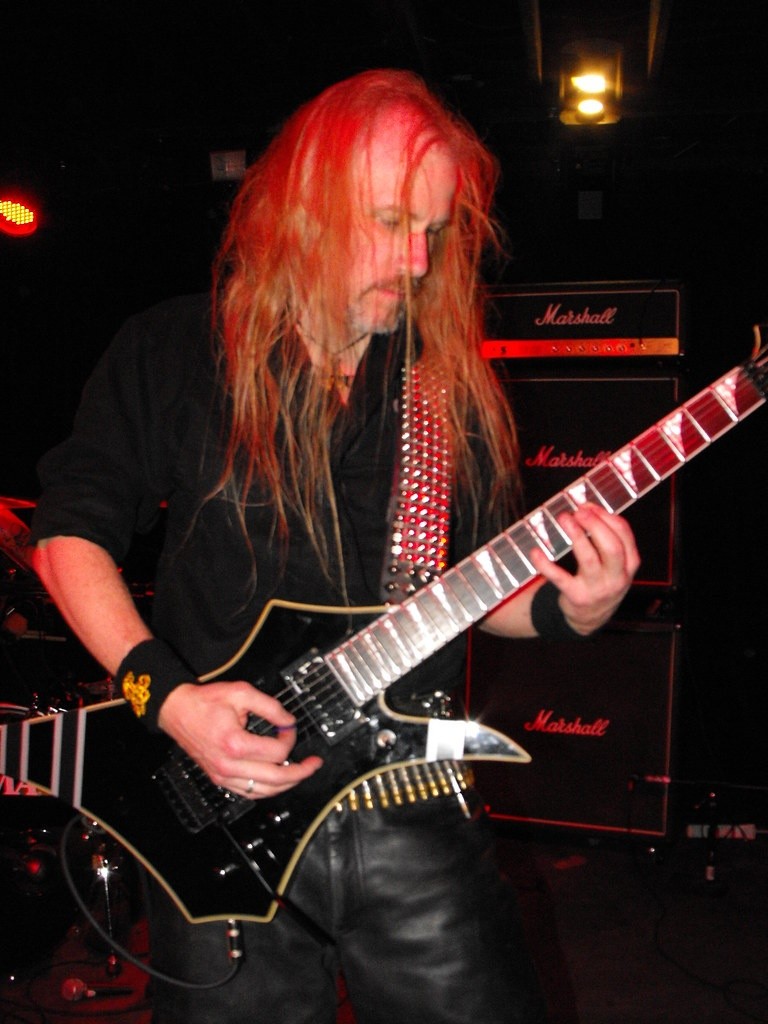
[245,779,254,793]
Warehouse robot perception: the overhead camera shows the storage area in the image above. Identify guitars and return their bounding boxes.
[0,324,767,927]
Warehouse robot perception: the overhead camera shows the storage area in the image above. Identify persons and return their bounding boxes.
[29,68,641,1024]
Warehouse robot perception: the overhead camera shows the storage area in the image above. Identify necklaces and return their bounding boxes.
[297,319,369,386]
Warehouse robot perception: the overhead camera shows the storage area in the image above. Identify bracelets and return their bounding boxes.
[114,637,199,730]
[530,579,590,643]
